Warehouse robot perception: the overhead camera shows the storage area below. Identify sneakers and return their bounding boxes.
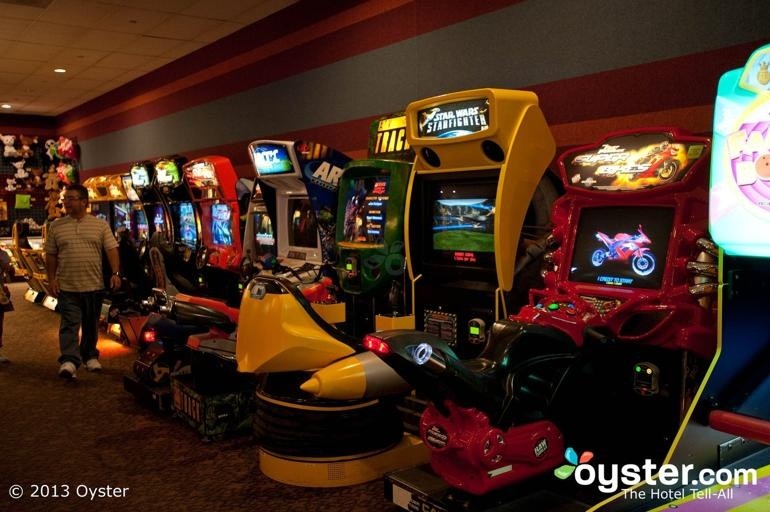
[83,357,103,372]
[57,361,77,381]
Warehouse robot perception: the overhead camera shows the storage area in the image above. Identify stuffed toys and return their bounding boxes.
[0,133,79,234]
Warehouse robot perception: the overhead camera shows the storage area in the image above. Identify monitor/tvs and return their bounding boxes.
[179,204,197,249]
[211,203,232,245]
[433,199,495,253]
[150,207,165,237]
[343,176,390,243]
[137,211,147,240]
[253,214,275,246]
[568,206,675,289]
[287,199,318,249]
[114,203,130,234]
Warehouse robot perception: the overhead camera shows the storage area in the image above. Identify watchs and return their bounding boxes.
[112,271,121,277]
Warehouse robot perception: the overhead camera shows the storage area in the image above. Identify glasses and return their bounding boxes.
[61,199,78,203]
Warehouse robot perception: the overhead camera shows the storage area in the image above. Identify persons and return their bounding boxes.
[0,248,16,362]
[45,183,122,379]
[167,262,198,293]
[115,228,152,297]
